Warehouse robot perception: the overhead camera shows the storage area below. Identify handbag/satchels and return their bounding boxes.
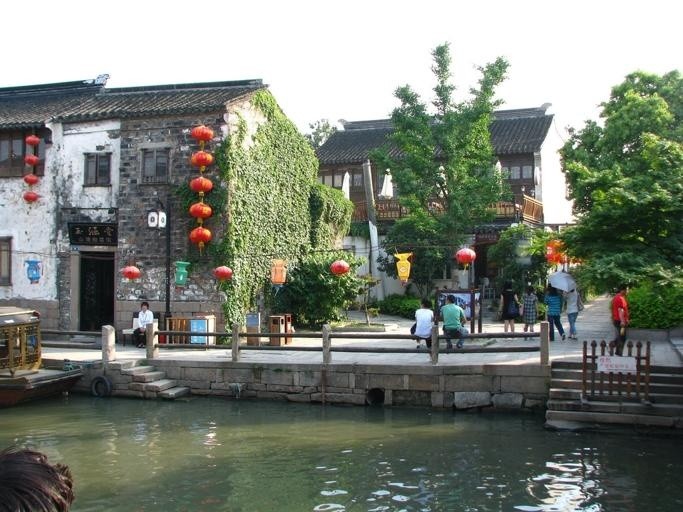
[577,293,584,311]
[519,303,525,316]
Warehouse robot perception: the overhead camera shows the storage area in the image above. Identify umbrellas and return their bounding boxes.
[548,271,578,293]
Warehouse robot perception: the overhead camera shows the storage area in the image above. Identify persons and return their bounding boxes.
[524,286,538,341]
[134,302,154,348]
[414,298,432,361]
[440,294,468,349]
[565,290,582,340]
[499,281,520,339]
[609,283,630,357]
[545,283,551,294]
[546,286,566,342]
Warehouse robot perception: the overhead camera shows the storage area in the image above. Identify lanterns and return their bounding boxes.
[328,257,351,277]
[270,258,288,290]
[23,133,41,207]
[174,260,191,287]
[456,244,481,274]
[547,239,582,265]
[189,123,214,255]
[394,250,413,286]
[122,266,142,284]
[214,264,234,284]
[23,257,44,285]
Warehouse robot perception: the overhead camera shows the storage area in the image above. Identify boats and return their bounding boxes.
[0,303,85,410]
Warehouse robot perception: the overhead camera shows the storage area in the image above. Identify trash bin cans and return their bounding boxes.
[268,313,293,347]
[189,314,216,351]
[247,312,261,347]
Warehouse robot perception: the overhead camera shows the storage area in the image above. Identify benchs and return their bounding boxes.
[122,311,160,347]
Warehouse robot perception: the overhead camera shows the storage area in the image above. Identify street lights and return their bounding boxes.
[146,195,174,347]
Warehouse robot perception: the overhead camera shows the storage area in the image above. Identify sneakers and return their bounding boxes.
[415,343,465,349]
[138,342,146,348]
[524,333,579,342]
[605,343,614,356]
